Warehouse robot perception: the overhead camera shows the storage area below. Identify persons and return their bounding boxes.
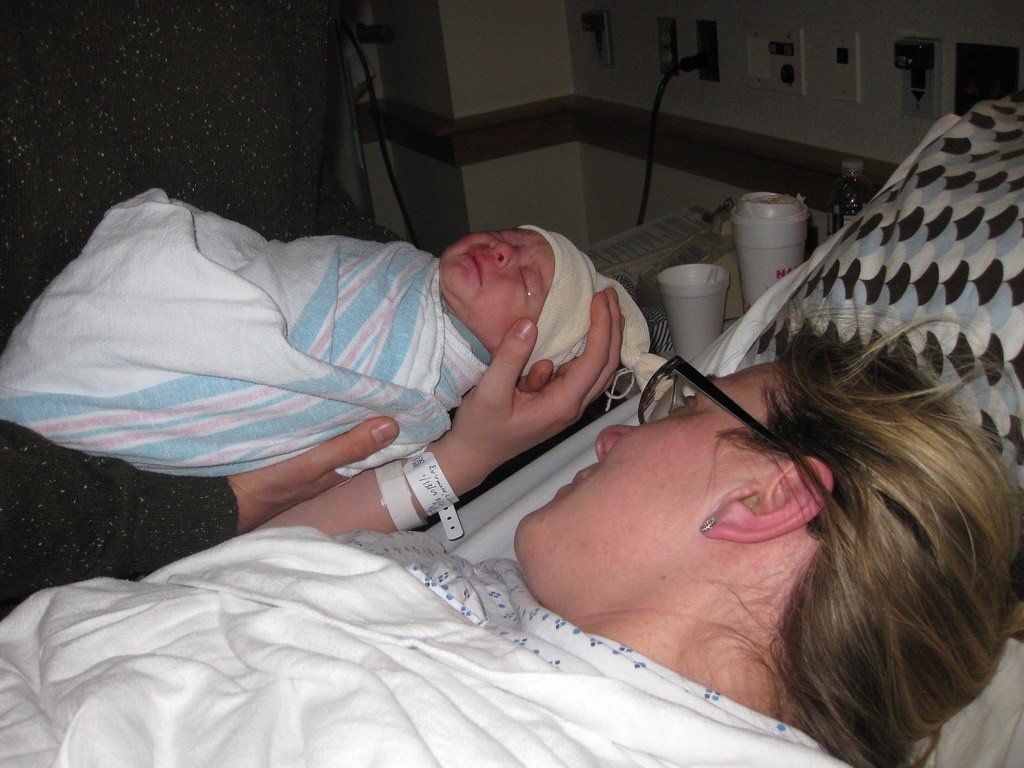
[252,286,1024,768]
[1,415,400,621]
[0,187,674,479]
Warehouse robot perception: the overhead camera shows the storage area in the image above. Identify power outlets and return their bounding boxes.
[900,37,943,120]
[589,10,612,65]
[695,19,720,83]
[655,16,679,79]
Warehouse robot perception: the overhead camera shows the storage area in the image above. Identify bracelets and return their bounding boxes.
[403,447,464,540]
[375,459,427,531]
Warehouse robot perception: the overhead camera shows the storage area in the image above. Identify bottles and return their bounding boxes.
[831,158,873,226]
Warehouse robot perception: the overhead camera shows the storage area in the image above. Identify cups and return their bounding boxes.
[729,191,811,315]
[656,262,730,363]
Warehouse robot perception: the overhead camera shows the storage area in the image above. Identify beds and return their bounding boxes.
[426,115,1024,768]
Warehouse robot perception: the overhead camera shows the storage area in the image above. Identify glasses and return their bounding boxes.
[637,356,849,515]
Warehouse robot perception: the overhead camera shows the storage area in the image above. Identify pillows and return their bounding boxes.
[736,96,1024,495]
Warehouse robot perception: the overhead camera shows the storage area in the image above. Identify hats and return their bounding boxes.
[517,225,676,402]
